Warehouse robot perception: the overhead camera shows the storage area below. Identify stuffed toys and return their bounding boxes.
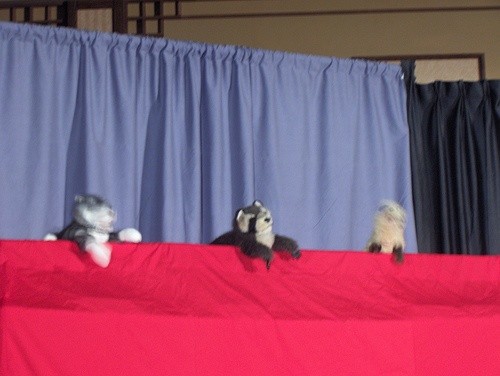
[365,199,408,262]
[42,193,144,268]
[209,199,301,271]
